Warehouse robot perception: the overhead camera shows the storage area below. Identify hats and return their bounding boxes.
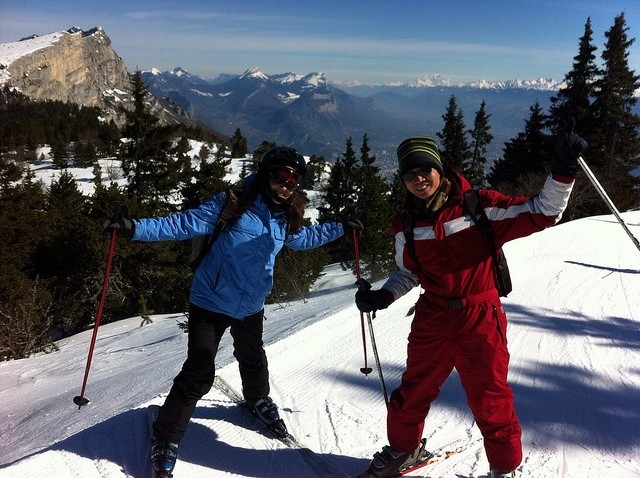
[397,136,445,176]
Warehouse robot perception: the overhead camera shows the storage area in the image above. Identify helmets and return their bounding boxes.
[260,146,306,192]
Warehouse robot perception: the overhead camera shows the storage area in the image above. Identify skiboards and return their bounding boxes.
[147,374,353,478]
[357,420,544,478]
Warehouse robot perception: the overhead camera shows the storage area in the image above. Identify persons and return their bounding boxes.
[100,144,367,474]
[354,116,590,478]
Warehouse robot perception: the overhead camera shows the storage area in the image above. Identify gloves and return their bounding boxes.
[101,217,135,236]
[548,117,589,177]
[342,217,364,237]
[356,288,395,319]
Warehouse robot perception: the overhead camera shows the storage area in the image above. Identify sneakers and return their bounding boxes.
[254,395,279,424]
[151,434,178,473]
[368,445,420,477]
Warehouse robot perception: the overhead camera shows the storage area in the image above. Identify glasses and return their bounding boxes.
[403,165,432,181]
[271,166,303,191]
